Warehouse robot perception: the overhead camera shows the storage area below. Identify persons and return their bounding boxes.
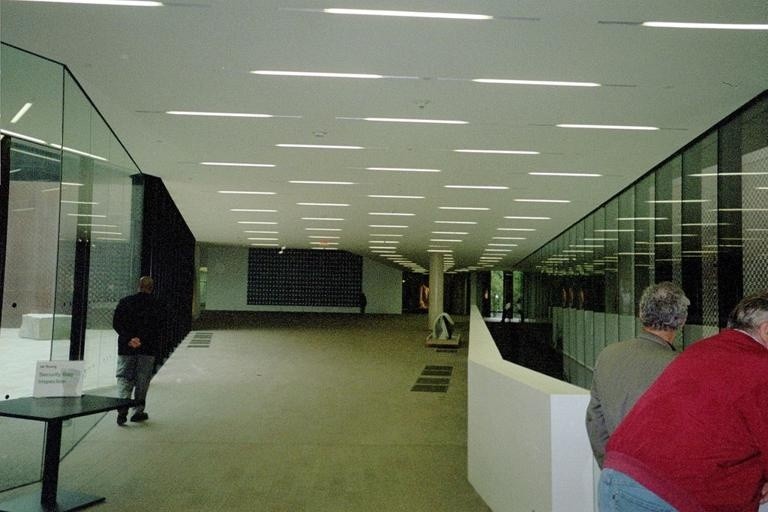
[111,275,165,426]
[585,280,691,472]
[594,290,768,511]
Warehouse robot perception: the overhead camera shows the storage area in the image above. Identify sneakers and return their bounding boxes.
[131,412,148,422]
[117,416,126,425]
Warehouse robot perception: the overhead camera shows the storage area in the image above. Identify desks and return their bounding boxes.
[0,392,147,512]
